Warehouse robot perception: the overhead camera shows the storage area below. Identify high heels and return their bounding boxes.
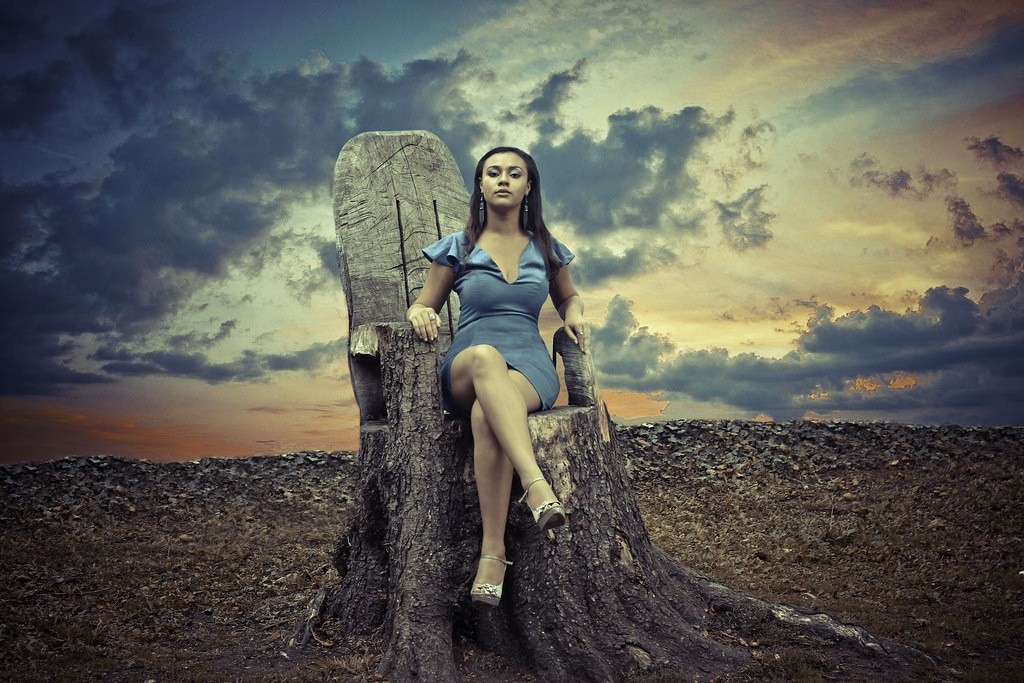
[470,555,513,606]
[518,478,566,540]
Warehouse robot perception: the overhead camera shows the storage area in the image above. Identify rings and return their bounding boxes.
[576,332,584,336]
[429,314,436,322]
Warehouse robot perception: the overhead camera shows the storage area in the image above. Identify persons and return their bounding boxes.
[406,146,587,605]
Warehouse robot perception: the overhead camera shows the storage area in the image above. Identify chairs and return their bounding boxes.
[299,130,887,683]
